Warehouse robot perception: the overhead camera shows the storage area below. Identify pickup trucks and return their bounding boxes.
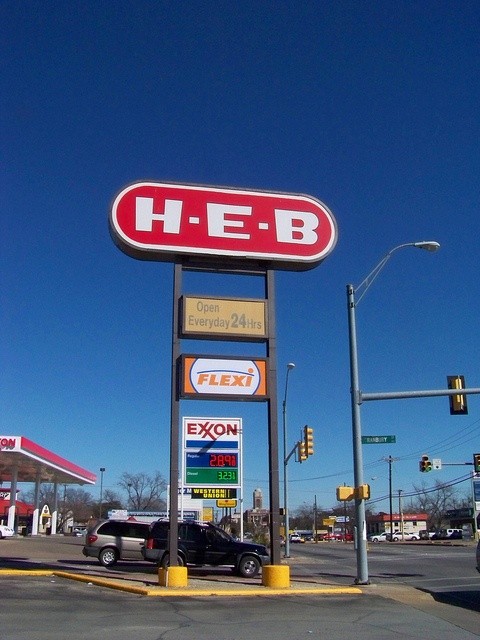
[280,530,353,548]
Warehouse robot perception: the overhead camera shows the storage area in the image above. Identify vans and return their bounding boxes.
[417,528,463,540]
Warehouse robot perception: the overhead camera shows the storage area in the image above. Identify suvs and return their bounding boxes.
[143,517,270,578]
[82,518,151,567]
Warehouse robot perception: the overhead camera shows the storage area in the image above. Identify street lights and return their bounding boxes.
[282,361,296,559]
[99,468,106,523]
[346,241,442,584]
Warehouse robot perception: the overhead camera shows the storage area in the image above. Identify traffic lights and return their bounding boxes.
[473,453,480,472]
[297,440,308,462]
[419,455,432,472]
[279,507,286,516]
[304,424,313,458]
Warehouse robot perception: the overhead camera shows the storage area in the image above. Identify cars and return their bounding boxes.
[244,532,254,539]
[0,525,15,539]
[392,530,421,541]
[370,531,394,543]
